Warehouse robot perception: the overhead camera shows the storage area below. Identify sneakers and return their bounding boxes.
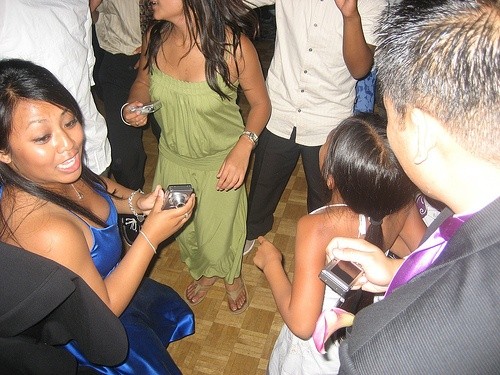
[120,216,141,247]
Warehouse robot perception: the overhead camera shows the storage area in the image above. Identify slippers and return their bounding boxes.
[183,276,219,306]
[225,274,249,315]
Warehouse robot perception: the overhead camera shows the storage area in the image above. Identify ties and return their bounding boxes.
[378,214,474,300]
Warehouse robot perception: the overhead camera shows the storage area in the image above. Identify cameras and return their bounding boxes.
[318,256,365,297]
[130,100,162,114]
[160,184,192,210]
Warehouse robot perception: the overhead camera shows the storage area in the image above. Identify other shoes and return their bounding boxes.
[242,238,256,256]
